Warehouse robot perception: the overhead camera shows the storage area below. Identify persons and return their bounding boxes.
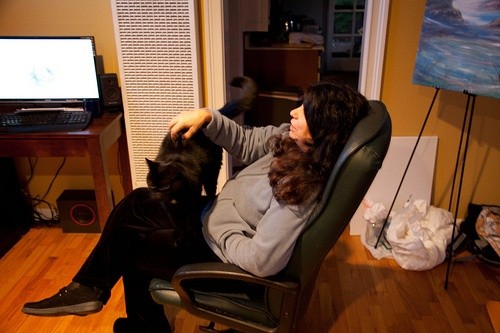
[22,82,371,333]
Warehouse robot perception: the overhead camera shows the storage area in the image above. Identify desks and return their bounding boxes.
[245,44,324,93]
[0,105,133,232]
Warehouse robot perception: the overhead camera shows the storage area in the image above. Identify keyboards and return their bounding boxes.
[0,111,91,132]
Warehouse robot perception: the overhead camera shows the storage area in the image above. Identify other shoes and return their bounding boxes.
[112,317,144,333]
[22,287,103,315]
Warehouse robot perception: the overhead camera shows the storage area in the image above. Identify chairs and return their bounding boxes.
[149,98,393,332]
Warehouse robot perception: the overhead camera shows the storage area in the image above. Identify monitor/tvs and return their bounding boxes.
[0,35,101,103]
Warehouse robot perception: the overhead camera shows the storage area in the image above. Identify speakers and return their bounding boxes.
[57,189,115,233]
[98,74,121,107]
